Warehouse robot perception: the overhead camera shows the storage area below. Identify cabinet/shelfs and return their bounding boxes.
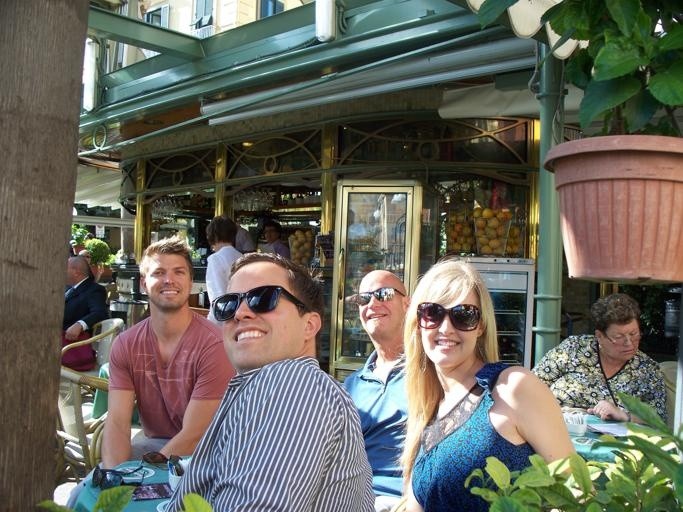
[152,195,321,243]
[110,263,332,371]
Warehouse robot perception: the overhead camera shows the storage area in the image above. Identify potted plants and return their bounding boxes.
[79,238,110,282]
[73,229,88,255]
[479,0,683,282]
[101,254,115,282]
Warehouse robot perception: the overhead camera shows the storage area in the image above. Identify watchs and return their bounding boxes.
[529,293,667,430]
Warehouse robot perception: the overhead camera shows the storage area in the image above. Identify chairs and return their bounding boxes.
[61,318,124,403]
[660,361,683,439]
[56,369,137,483]
[561,308,591,341]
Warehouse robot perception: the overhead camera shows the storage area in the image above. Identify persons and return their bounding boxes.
[206,216,243,325]
[235,224,255,253]
[65,237,235,510]
[343,269,410,498]
[399,261,576,512]
[260,221,290,258]
[162,255,373,512]
[79,250,104,283]
[623,408,631,422]
[64,256,106,340]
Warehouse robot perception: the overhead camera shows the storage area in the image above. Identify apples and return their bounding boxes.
[289,229,314,264]
[448,207,522,255]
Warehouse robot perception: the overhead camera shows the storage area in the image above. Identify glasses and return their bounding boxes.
[417,302,482,331]
[90,467,144,489]
[601,329,642,346]
[213,285,304,322]
[144,451,183,477]
[355,287,406,305]
